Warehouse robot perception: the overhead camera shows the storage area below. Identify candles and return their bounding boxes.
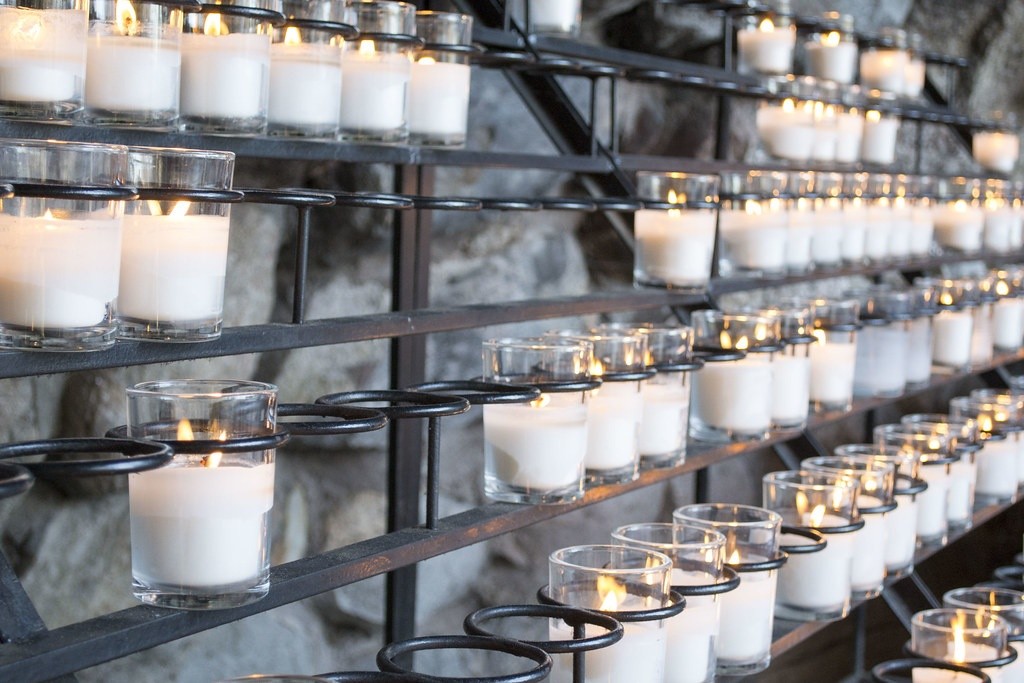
[0,0,1023,682]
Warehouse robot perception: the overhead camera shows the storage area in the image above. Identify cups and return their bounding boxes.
[0,1,475,150]
[125,379,279,611]
[0,139,128,353]
[483,0,1024,683]
[116,144,235,343]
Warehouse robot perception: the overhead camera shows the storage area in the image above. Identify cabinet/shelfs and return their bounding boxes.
[0,0,1024,683]
